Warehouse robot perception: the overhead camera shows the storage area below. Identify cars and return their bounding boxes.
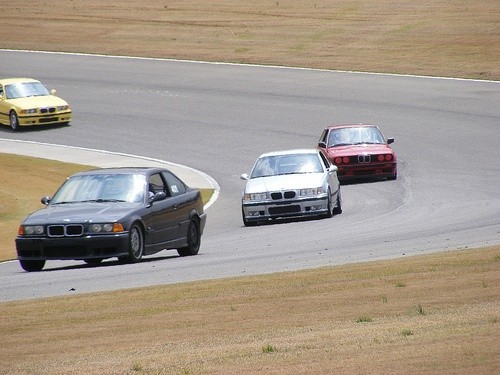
[318,124,398,184]
[0,77,71,130]
[15,167,207,273]
[239,148,344,228]
